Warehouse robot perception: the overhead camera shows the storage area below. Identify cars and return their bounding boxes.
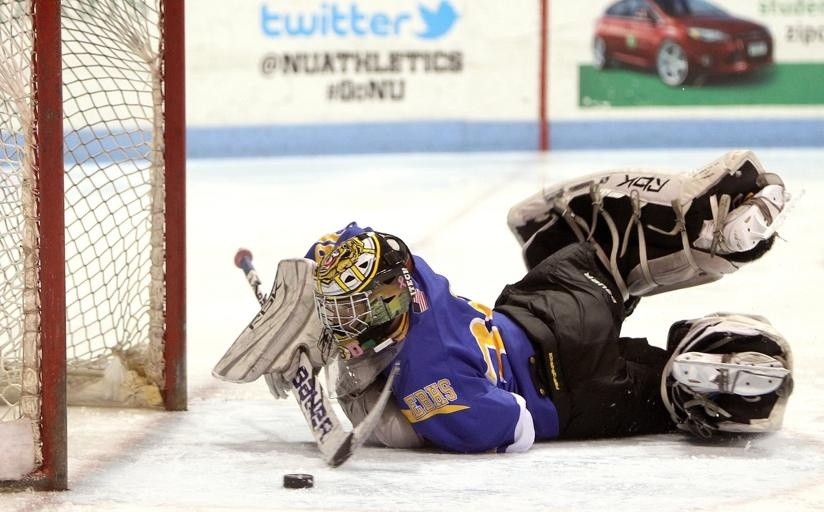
[588,0,773,87]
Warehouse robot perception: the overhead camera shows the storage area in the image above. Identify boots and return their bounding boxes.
[672,351,791,397]
[692,184,785,255]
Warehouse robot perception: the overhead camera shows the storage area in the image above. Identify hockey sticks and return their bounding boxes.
[234,250,401,467]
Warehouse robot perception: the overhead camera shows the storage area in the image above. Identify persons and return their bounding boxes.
[263,184,785,453]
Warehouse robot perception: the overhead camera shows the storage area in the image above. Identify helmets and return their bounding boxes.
[313,231,415,364]
